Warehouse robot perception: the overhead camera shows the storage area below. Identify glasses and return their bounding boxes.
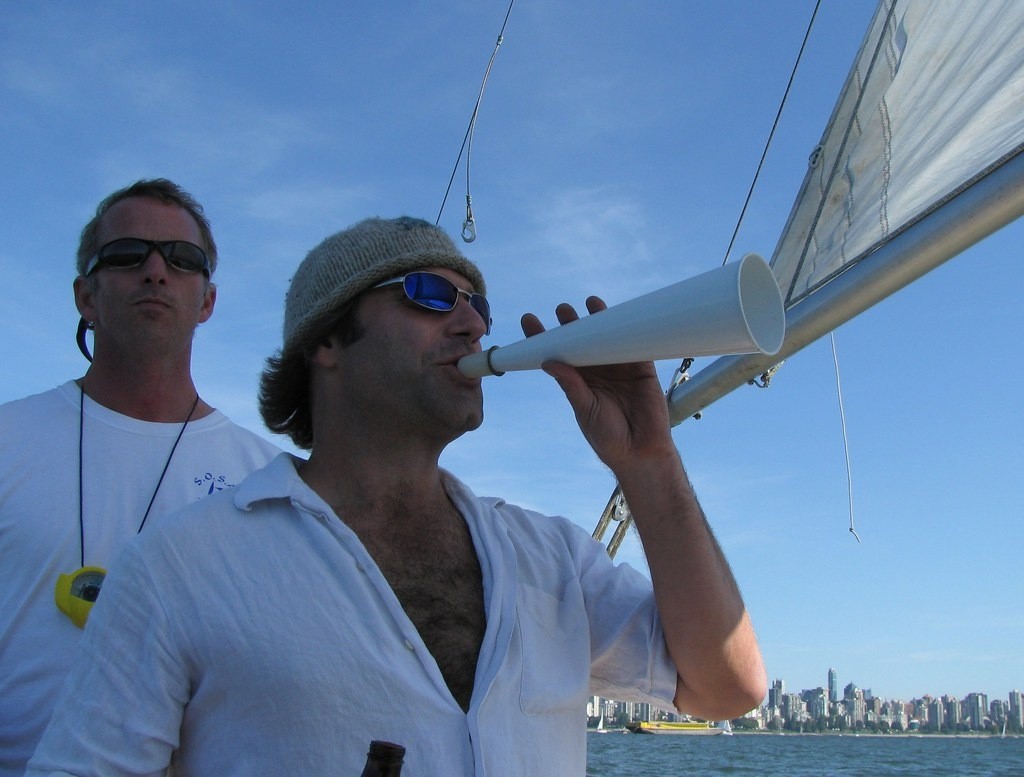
[82,236,214,278]
[368,270,492,336]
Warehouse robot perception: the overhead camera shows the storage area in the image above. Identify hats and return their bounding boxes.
[283,215,488,361]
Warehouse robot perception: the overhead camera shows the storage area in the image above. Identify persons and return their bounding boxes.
[0,178,286,776]
[21,216,768,777]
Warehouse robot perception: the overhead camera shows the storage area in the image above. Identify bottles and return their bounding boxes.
[360,740,407,777]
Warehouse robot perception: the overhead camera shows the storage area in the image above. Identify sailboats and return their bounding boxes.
[719,720,733,736]
[596,707,608,734]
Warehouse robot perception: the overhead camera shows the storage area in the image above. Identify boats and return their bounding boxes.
[624,713,725,735]
